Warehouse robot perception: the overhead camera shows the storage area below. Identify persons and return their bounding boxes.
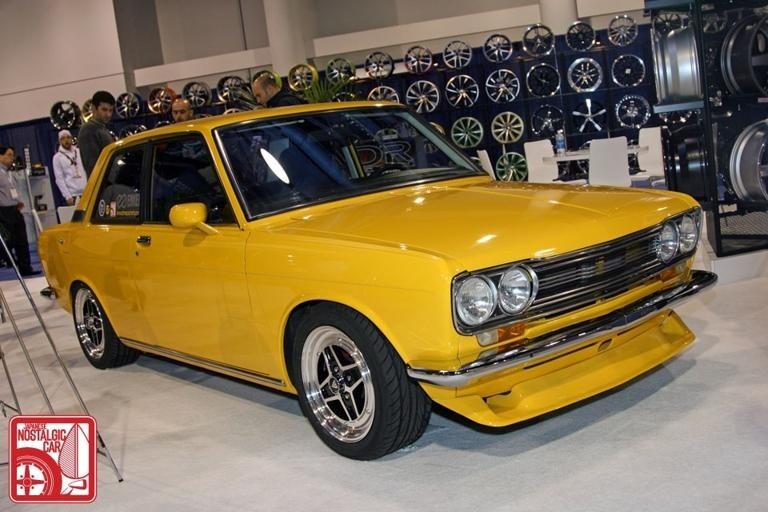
[79,90,116,181]
[172,99,193,122]
[251,72,309,109]
[0,146,43,276]
[53,130,87,202]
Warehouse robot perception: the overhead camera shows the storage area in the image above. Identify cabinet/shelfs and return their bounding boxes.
[645,0,768,259]
[16,164,56,244]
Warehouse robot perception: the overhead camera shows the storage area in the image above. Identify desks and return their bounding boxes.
[542,145,649,162]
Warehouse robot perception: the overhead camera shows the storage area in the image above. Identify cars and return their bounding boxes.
[37,100,702,463]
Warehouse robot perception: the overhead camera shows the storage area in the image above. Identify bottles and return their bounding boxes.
[555,130,566,160]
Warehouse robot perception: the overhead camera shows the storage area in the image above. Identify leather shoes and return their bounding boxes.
[19,270,44,278]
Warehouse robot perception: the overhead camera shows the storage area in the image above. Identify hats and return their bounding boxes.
[59,130,72,140]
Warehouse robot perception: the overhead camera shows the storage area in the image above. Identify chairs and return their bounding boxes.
[524,139,586,186]
[581,136,631,187]
[476,150,496,180]
[629,126,666,187]
[57,206,77,224]
